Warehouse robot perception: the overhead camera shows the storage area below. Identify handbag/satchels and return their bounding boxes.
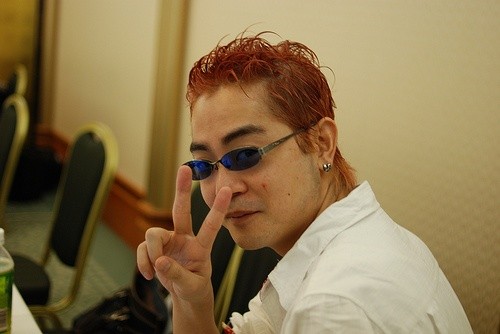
[71,286,167,334]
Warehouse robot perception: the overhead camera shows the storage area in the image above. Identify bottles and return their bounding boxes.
[0,227,15,334]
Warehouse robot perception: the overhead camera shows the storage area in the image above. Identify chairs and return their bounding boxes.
[3,63,29,96]
[10,121,118,334]
[127,183,245,334]
[0,95,30,222]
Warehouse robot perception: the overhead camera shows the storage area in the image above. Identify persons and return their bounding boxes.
[137,37,474,334]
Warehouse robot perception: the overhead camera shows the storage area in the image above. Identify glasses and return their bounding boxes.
[180,118,323,181]
